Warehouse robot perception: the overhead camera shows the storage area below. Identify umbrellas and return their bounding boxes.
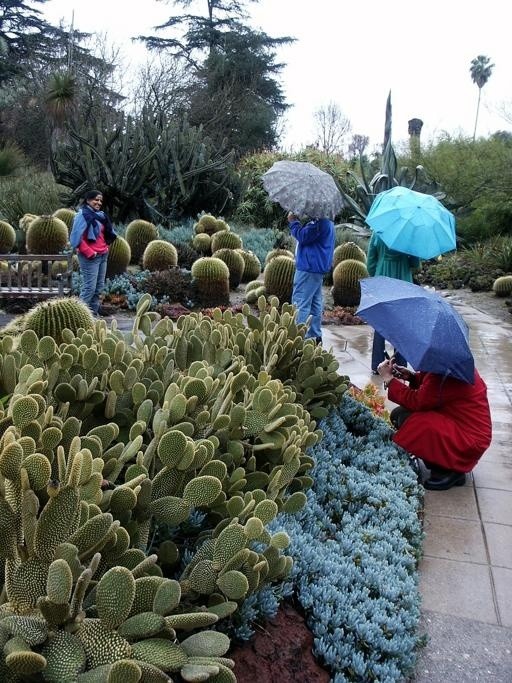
[353,274,475,384]
[364,185,458,260]
[260,159,345,222]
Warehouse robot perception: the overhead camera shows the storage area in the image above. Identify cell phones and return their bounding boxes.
[393,365,403,375]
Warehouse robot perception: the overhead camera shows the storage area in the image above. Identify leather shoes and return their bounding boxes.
[424,470,467,490]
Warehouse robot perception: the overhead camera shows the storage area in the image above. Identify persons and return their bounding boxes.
[286,212,337,346]
[377,356,493,491]
[366,231,421,376]
[67,189,118,317]
